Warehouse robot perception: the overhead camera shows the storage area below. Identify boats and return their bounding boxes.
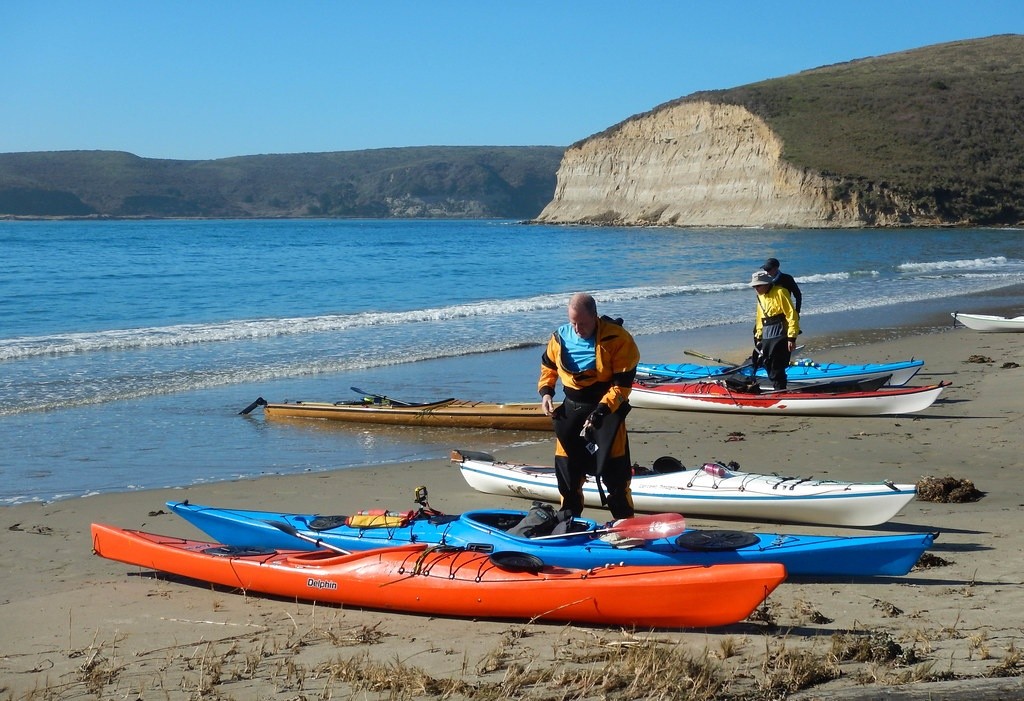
[83,524,788,629]
[631,374,952,415]
[950,301,1024,332]
[633,346,925,387]
[256,392,576,432]
[438,443,919,529]
[160,481,943,575]
[697,366,893,392]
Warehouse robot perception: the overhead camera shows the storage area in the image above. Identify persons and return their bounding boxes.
[538,293,640,527]
[748,271,799,391]
[754,258,802,367]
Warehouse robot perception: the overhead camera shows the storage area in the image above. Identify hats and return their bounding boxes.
[748,271,774,287]
[759,257,780,271]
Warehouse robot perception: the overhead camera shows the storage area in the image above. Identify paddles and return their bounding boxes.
[720,344,806,374]
[529,512,686,540]
[684,349,739,366]
[350,387,411,405]
[264,520,351,556]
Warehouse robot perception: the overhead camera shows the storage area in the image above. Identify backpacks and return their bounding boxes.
[504,499,590,539]
